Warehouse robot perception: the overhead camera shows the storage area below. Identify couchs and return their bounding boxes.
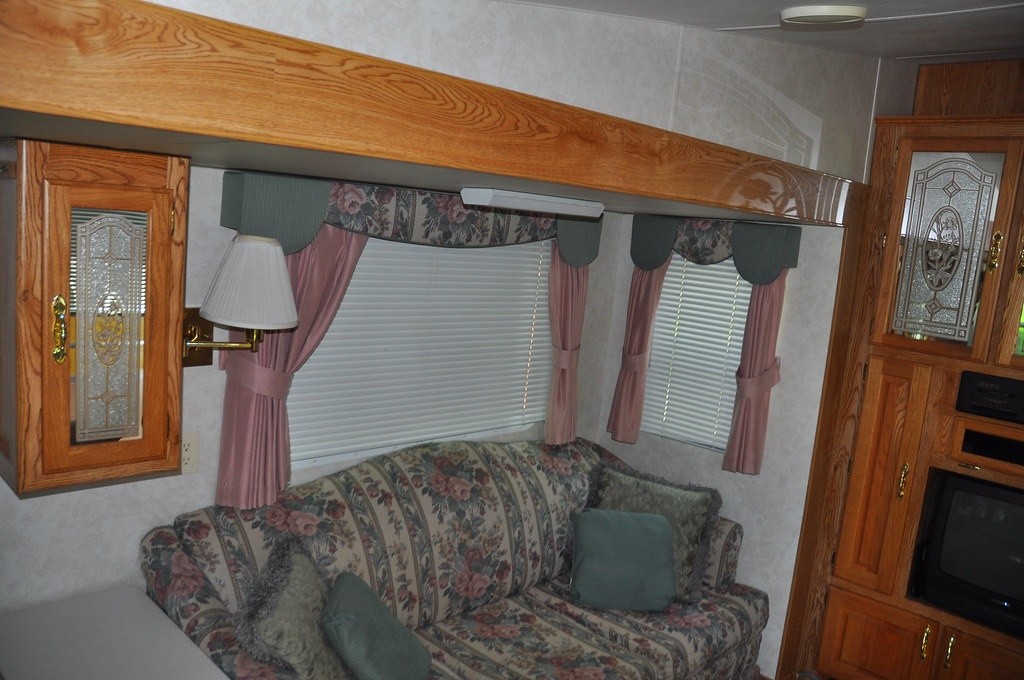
[142,421,771,680]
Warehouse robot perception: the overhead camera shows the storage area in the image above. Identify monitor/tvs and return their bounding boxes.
[916,476,1024,641]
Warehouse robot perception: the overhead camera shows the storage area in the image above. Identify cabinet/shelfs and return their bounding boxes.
[795,114,1024,680]
[0,140,191,498]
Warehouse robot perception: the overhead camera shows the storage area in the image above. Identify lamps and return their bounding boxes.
[182,234,299,368]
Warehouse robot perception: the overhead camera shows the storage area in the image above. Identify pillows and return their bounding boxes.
[584,460,724,607]
[322,574,431,680]
[231,533,353,680]
[568,507,689,615]
